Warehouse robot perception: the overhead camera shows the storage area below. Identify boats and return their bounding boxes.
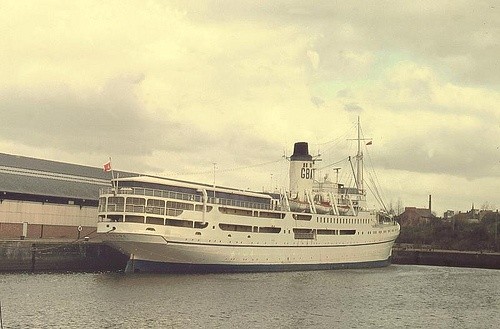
[314,202,334,214]
[286,191,309,212]
[337,205,350,213]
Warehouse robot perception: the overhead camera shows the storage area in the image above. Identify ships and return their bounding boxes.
[96,115,402,275]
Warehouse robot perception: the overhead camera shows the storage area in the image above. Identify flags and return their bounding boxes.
[104,162,111,171]
